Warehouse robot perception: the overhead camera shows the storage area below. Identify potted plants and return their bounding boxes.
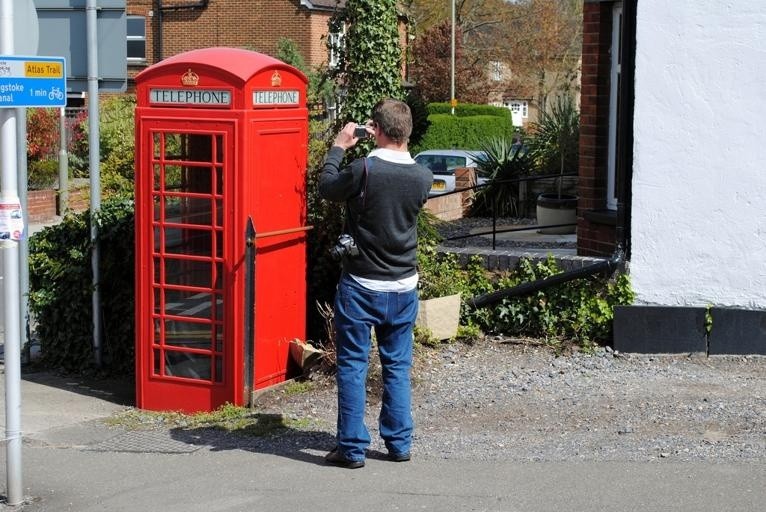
[414,263,461,341]
[527,93,581,234]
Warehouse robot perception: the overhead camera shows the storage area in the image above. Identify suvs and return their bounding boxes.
[412,149,495,198]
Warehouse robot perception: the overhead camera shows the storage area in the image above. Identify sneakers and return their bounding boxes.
[325,447,364,468]
[390,453,410,462]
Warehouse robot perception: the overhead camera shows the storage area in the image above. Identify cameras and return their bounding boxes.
[327,232,360,261]
[353,127,370,138]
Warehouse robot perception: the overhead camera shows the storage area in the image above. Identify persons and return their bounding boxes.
[319,100,433,468]
